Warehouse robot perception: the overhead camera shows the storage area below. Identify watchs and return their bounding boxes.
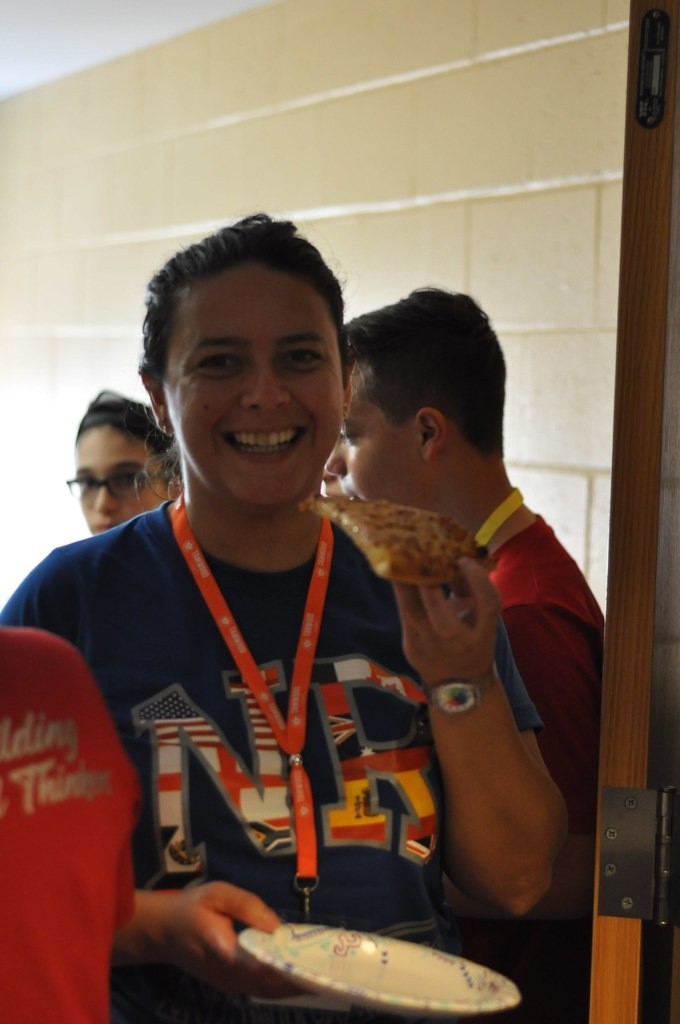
[424,673,502,716]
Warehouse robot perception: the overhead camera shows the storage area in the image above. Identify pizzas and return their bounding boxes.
[299,496,500,583]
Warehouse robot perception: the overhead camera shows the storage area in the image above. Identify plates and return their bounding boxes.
[238,922,523,1022]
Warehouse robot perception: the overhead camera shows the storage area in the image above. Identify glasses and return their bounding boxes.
[68,473,159,500]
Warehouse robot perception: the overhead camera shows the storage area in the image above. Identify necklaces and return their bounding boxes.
[475,488,523,551]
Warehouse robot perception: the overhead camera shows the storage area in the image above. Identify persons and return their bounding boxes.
[0,626,144,1024]
[0,213,567,1024]
[318,286,605,1024]
[66,391,182,537]
